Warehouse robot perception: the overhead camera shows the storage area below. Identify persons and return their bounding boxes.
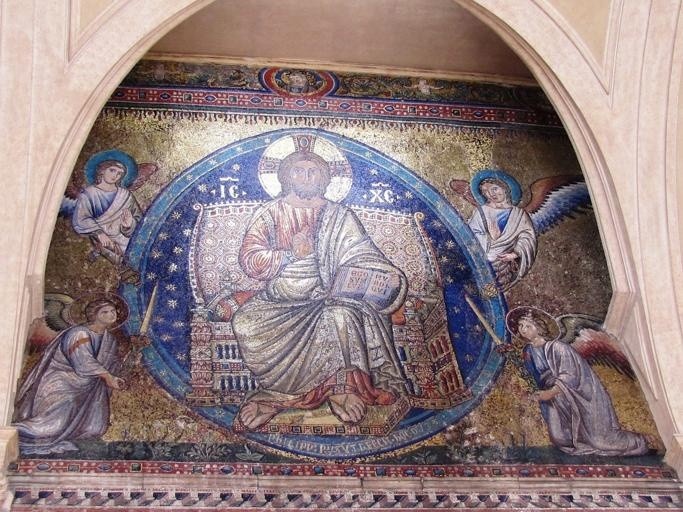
[234,150,409,431]
[136,63,180,81]
[451,167,590,290]
[494,305,663,459]
[403,78,445,95]
[281,72,317,93]
[58,150,157,264]
[12,292,142,448]
